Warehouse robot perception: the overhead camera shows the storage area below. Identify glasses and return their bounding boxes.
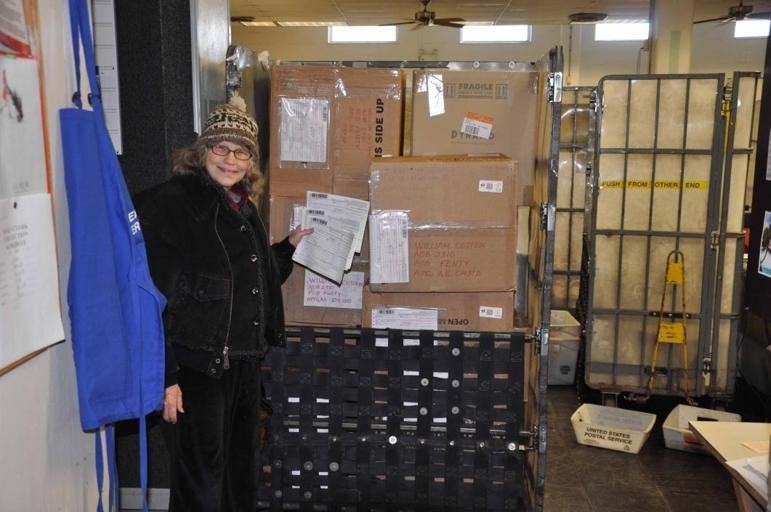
[207,144,256,162]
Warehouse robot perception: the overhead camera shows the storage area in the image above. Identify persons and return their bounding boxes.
[130,91,315,511]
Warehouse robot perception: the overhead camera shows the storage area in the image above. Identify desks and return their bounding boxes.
[686,421,771,512]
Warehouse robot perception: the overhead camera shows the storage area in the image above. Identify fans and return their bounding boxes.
[380,1,465,31]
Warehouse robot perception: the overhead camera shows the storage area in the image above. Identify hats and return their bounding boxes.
[200,91,260,149]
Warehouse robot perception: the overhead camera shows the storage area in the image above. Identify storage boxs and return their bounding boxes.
[412,70,541,207]
[362,282,517,331]
[266,194,370,328]
[370,154,521,292]
[266,65,405,199]
